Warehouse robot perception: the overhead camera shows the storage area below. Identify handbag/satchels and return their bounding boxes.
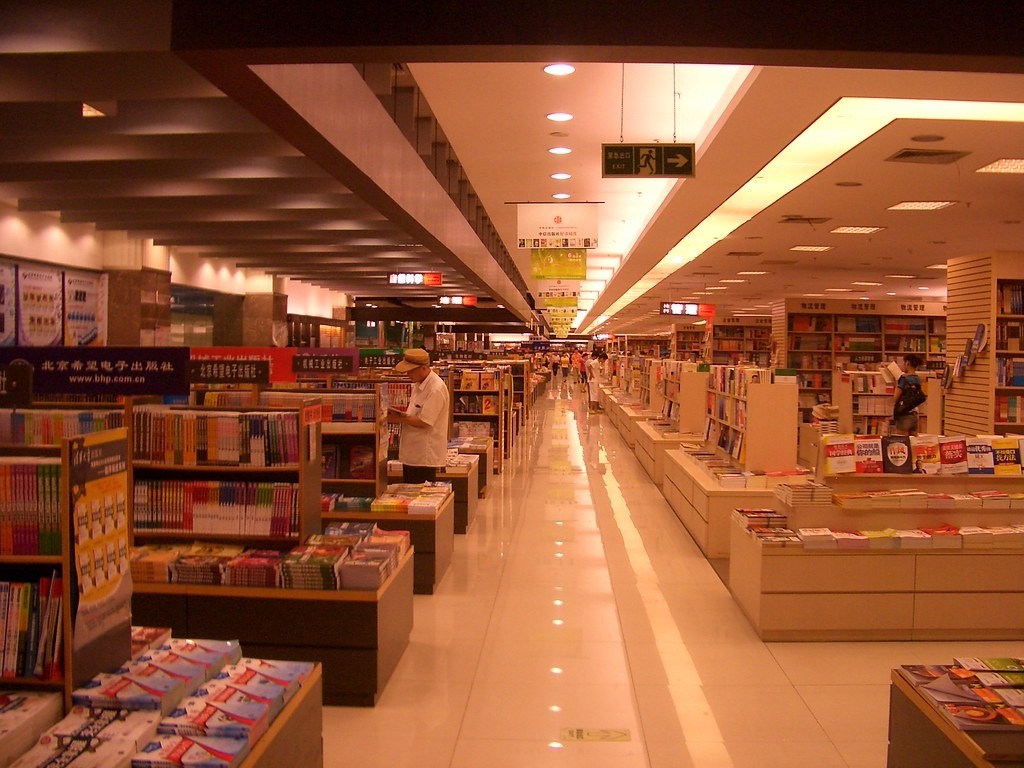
[893,375,927,417]
[546,362,550,365]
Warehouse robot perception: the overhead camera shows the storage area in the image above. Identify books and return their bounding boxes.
[320,440,453,515]
[727,431,1024,555]
[130,520,412,591]
[1,577,66,686]
[785,278,1024,434]
[0,624,319,768]
[133,409,300,467]
[0,462,72,557]
[132,478,304,538]
[596,327,815,490]
[195,391,380,422]
[0,408,125,448]
[886,656,1023,732]
[329,366,510,475]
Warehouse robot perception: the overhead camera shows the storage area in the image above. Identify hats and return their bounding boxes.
[396,349,429,372]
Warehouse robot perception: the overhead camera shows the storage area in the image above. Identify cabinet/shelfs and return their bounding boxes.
[588,254,1024,768]
[0,349,552,768]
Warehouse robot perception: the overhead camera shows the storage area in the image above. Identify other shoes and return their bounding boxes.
[590,410,595,414]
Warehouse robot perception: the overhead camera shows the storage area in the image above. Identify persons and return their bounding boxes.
[385,347,450,482]
[892,353,921,436]
[542,347,608,416]
